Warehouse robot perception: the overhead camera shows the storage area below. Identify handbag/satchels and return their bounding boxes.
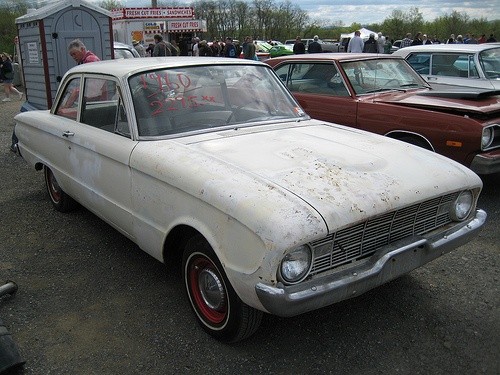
[5,71,15,79]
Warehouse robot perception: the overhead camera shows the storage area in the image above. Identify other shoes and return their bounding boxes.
[18,92,23,101]
[2,98,11,102]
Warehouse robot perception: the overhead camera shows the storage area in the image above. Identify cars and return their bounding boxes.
[10,54,490,344]
[328,42,500,95]
[189,51,500,175]
[113,41,141,59]
[252,38,292,54]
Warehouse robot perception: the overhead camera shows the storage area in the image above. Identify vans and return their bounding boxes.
[283,38,325,51]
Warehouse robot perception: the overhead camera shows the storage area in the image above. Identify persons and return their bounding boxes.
[131,39,147,57]
[377,32,387,54]
[0,52,22,102]
[308,35,322,54]
[384,36,392,54]
[146,34,259,60]
[293,35,305,55]
[362,34,379,53]
[268,40,277,46]
[345,37,351,53]
[347,31,364,53]
[68,38,100,65]
[400,32,496,48]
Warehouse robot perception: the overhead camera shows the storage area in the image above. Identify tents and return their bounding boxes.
[338,28,380,51]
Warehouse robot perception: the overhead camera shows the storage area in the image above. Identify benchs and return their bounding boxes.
[414,64,458,74]
[84,100,176,126]
[282,77,325,90]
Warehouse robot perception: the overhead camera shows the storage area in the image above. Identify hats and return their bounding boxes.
[131,40,139,45]
[199,40,208,44]
[194,38,200,41]
